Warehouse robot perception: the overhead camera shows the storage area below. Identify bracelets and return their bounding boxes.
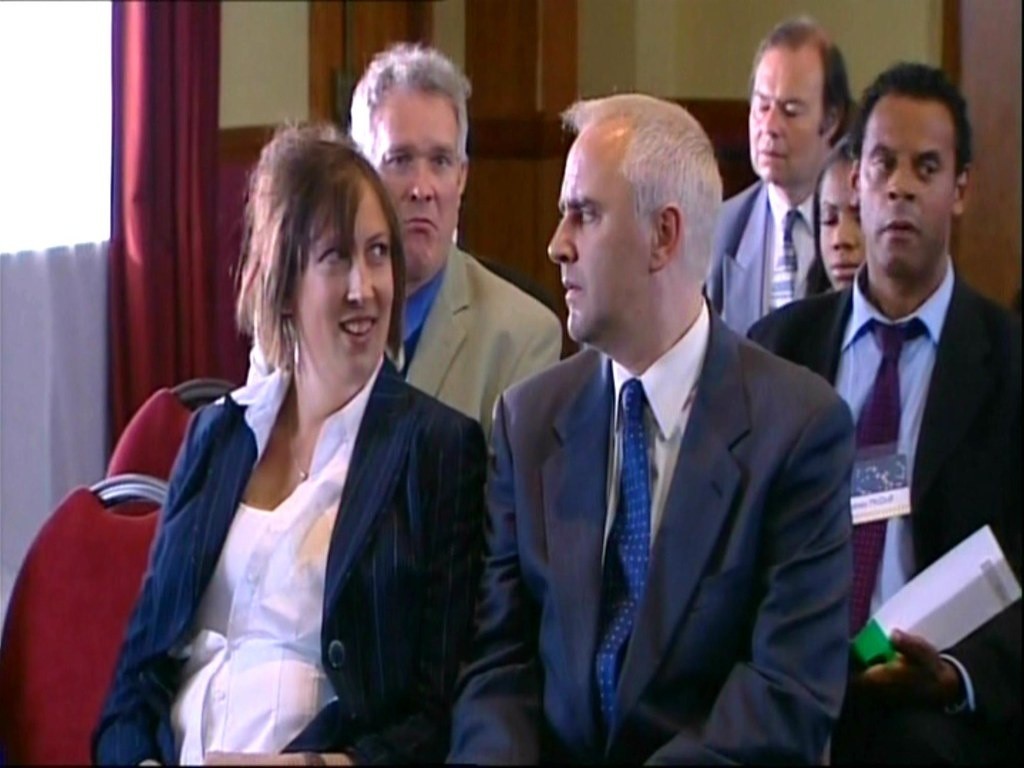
[305,753,326,766]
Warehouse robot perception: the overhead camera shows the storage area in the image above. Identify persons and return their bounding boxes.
[446,93,857,768]
[696,18,849,336]
[349,50,562,439]
[803,137,867,295]
[745,61,1024,768]
[90,138,489,766]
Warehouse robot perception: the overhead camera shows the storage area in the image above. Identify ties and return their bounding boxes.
[592,378,651,746]
[849,317,928,640]
[769,210,803,312]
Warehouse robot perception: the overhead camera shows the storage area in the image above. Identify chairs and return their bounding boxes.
[0,374,237,768]
